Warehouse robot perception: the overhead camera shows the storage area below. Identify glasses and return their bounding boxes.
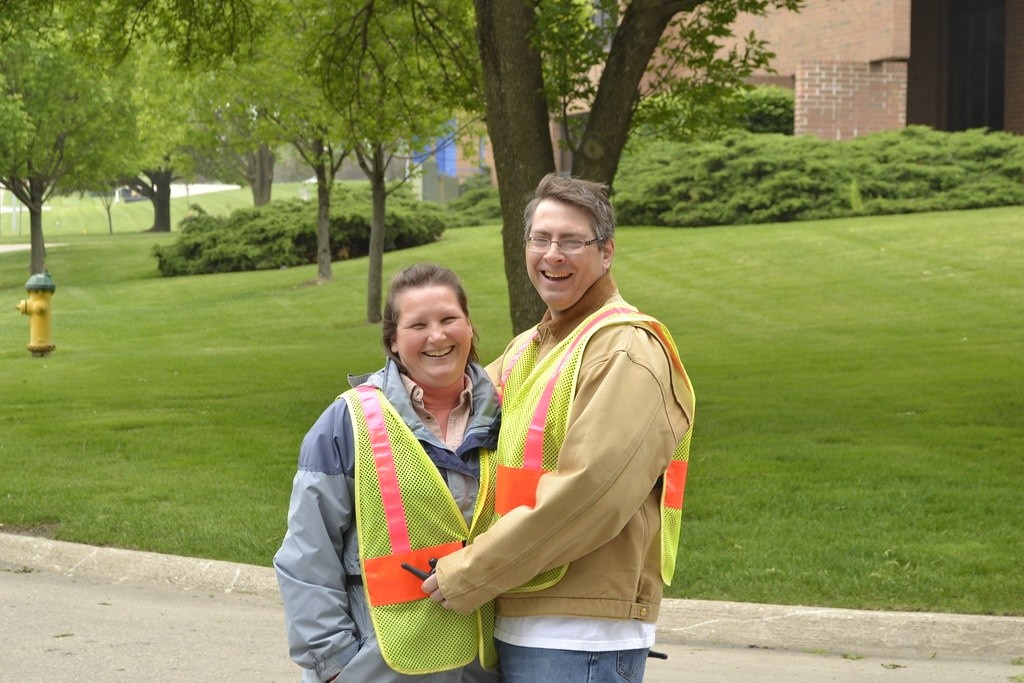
[523,235,606,253]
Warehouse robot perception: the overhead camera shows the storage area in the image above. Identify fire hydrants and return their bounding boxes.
[14,268,57,357]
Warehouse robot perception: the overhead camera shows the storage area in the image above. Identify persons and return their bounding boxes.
[271,259,502,683]
[421,171,697,682]
